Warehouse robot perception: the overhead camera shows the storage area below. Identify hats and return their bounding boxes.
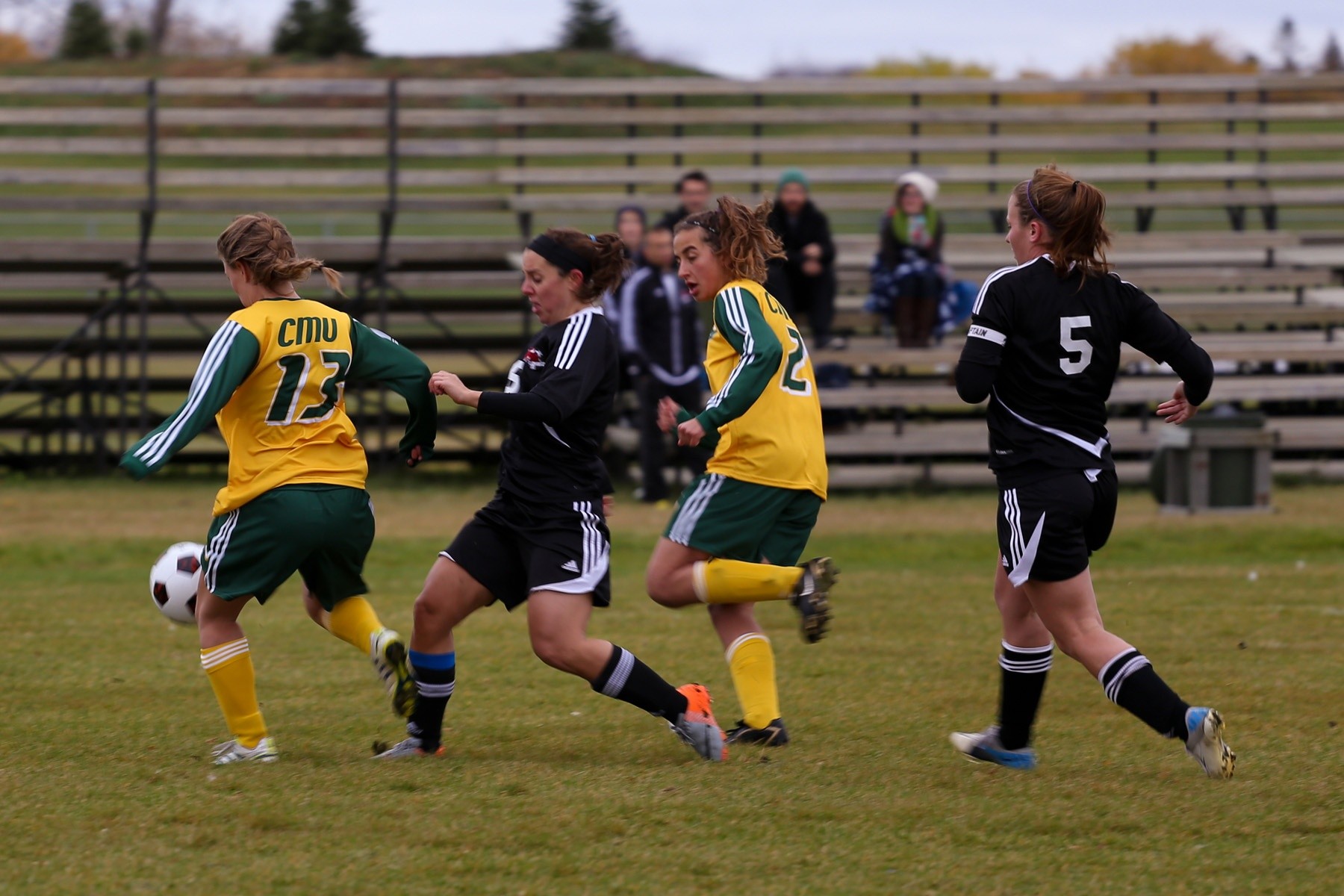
[776,169,810,195]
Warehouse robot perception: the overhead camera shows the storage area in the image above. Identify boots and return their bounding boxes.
[897,296,915,346]
[918,297,939,346]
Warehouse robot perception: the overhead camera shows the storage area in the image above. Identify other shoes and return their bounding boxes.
[650,499,671,511]
[602,495,614,516]
[812,336,844,349]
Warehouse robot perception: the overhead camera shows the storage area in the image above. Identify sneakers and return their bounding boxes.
[790,556,841,645]
[948,727,1034,772]
[209,736,276,762]
[371,627,416,719]
[672,683,728,761]
[1184,707,1237,781]
[721,719,789,748]
[369,737,443,760]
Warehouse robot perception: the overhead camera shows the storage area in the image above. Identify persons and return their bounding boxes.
[946,164,1238,784]
[118,209,436,765]
[618,223,713,506]
[601,205,649,494]
[630,191,840,745]
[643,169,716,232]
[359,225,734,762]
[867,168,950,349]
[746,170,848,350]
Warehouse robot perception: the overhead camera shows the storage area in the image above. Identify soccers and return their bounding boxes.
[148,541,209,626]
